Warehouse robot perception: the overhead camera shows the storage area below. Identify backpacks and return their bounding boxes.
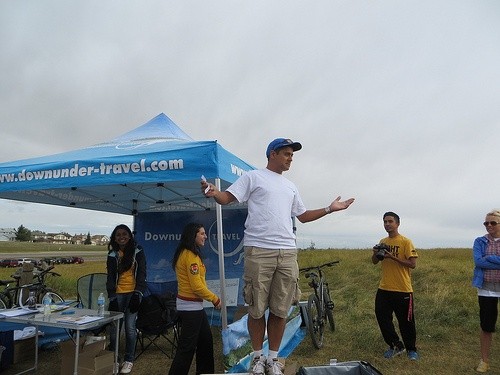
[136,297,178,335]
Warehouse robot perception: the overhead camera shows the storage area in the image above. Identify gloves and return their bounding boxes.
[128,291,140,314]
[108,296,119,312]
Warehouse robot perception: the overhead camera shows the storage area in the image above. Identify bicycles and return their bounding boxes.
[299,261,340,350]
[0,265,66,309]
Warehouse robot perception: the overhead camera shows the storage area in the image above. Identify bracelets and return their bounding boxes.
[325,206,332,214]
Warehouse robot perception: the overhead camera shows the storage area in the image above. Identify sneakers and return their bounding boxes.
[120,361,133,374]
[113,362,120,375]
[266,358,284,375]
[251,354,267,375]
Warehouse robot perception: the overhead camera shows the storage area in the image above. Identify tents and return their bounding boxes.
[0,113,271,375]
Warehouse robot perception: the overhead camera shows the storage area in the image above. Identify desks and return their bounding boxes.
[0,303,124,375]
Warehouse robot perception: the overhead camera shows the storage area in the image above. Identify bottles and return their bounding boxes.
[29,291,37,309]
[97,293,105,317]
[43,293,53,317]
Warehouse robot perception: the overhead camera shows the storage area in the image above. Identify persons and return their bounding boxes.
[168,223,221,375]
[372,212,418,360]
[201,138,354,375]
[106,224,146,374]
[472,210,500,372]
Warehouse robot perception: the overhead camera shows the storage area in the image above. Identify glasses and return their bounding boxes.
[272,139,293,149]
[483,221,500,226]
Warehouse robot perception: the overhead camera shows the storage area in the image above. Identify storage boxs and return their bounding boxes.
[0,330,34,363]
[60,335,114,375]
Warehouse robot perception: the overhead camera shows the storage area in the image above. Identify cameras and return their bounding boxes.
[375,243,389,260]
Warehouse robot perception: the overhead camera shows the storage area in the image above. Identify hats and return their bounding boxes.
[266,137,302,159]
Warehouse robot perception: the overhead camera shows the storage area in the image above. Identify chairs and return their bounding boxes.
[70,273,111,350]
[133,292,180,360]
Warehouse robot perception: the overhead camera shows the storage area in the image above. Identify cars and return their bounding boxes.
[0,256,84,268]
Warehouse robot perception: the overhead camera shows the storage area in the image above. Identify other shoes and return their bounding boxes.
[476,359,490,372]
[384,346,406,359]
[407,351,419,361]
